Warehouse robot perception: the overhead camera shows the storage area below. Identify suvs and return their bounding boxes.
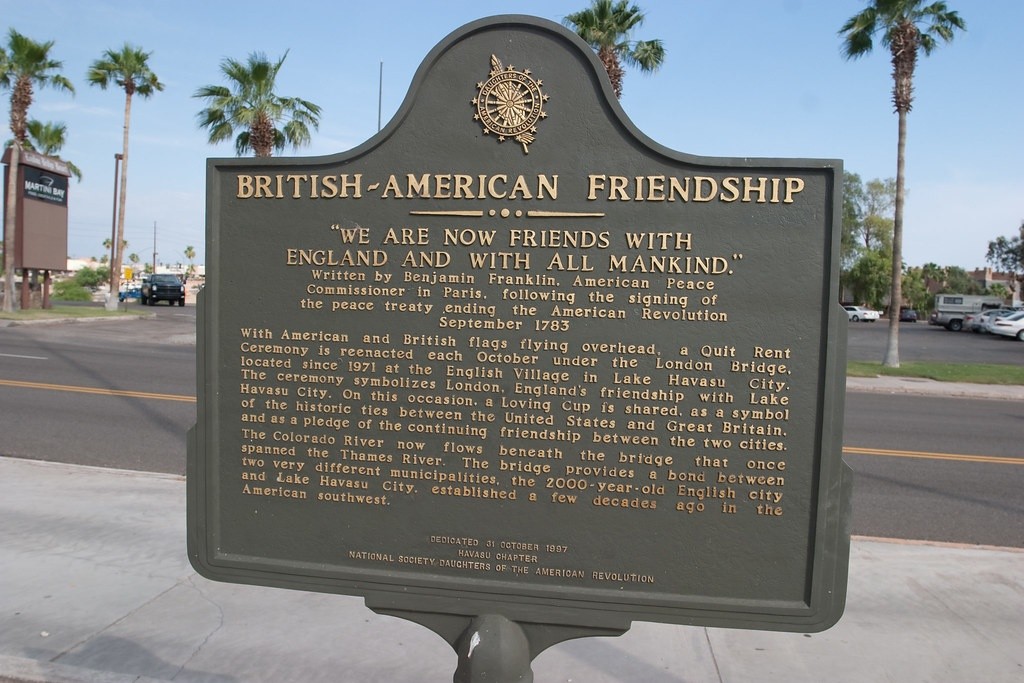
[140,272,187,307]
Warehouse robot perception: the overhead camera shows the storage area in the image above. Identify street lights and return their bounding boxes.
[109,153,124,293]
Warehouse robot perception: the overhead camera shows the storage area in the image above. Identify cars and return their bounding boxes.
[843,305,880,322]
[962,302,1024,341]
[899,308,916,323]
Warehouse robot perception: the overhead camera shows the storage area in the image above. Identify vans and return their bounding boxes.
[929,293,1005,332]
